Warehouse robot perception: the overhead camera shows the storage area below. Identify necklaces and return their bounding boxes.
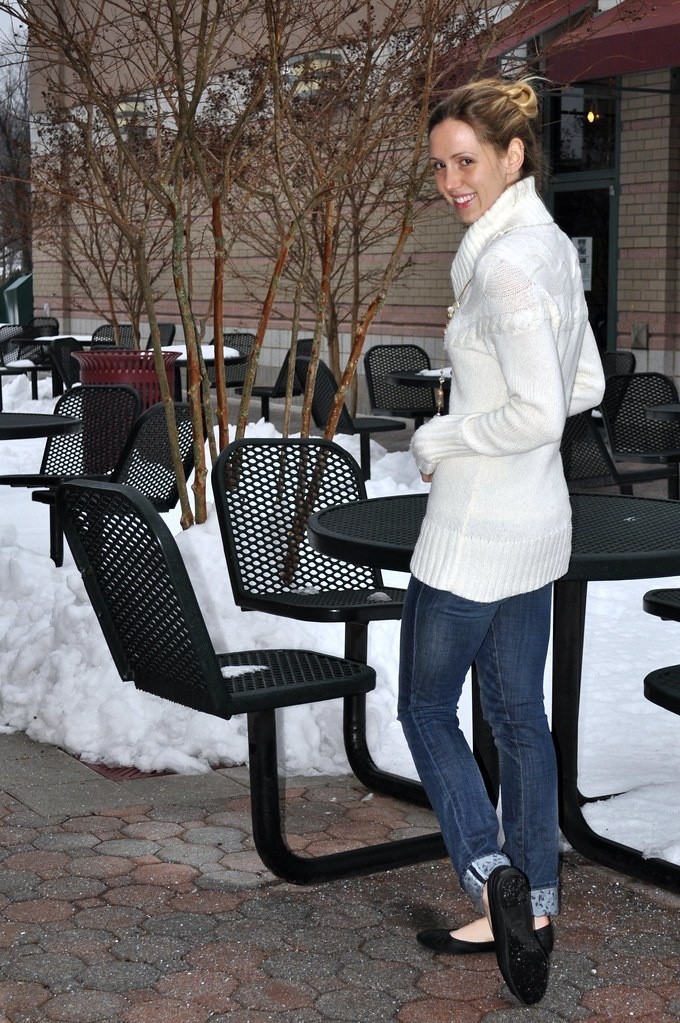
[434,276,474,415]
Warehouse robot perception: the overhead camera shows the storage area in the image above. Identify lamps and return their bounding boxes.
[588,109,604,123]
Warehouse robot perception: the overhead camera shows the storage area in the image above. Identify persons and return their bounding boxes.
[397,73,606,1004]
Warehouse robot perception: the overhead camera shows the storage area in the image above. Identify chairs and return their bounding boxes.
[0,316,680,721]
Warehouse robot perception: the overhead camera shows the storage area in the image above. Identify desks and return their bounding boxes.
[385,369,451,434]
[33,336,115,399]
[643,403,680,502]
[140,344,242,405]
[0,411,82,441]
[245,491,680,898]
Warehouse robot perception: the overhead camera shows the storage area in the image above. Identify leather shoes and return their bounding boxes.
[486,864,548,1006]
[416,920,558,954]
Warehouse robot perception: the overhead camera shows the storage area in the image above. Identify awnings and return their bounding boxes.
[436,0,597,80]
[546,0,680,85]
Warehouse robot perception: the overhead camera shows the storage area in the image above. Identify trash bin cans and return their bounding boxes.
[3,273,33,325]
[70,350,183,475]
